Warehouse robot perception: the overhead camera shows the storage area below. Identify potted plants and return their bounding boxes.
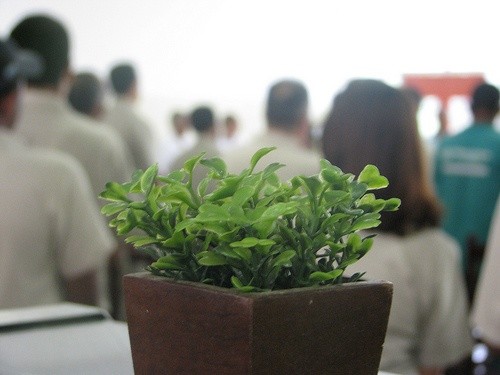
[98,146,401,375]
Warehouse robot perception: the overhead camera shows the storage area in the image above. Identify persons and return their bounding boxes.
[322,79,499,375]
[70,64,323,204]
[0,33,119,314]
[7,14,135,312]
